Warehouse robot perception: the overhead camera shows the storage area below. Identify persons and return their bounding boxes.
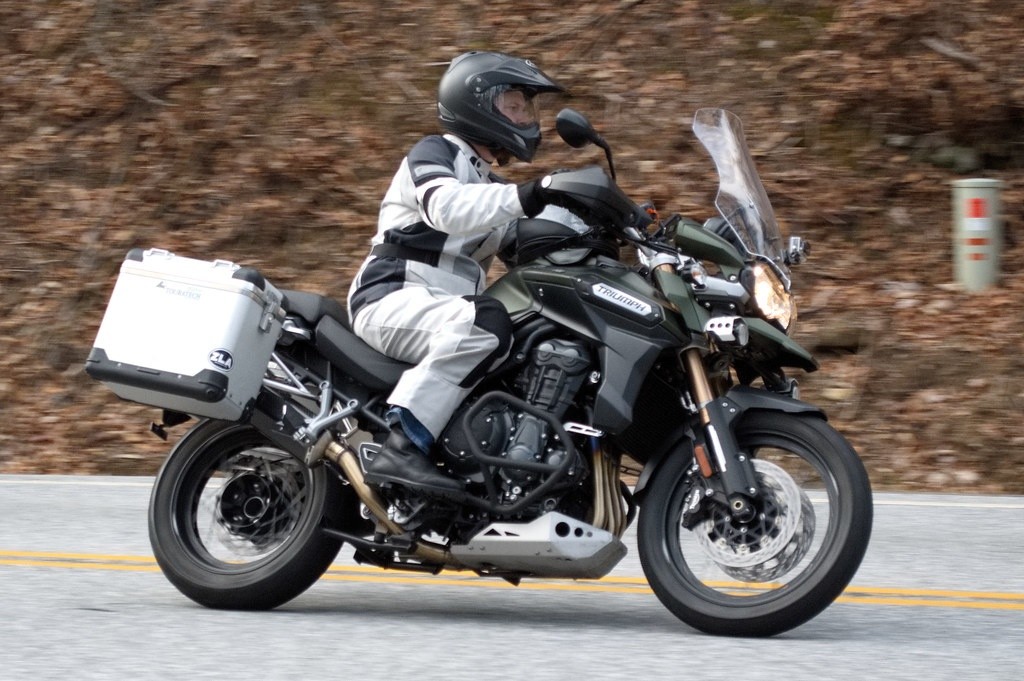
[346,50,589,500]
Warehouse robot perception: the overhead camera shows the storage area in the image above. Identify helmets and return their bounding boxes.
[436,50,565,162]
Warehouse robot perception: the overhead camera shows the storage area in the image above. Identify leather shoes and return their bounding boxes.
[362,428,463,501]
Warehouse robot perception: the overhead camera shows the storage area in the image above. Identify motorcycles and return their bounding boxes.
[86,108,874,636]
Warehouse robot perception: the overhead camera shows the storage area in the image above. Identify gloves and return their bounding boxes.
[516,166,576,218]
